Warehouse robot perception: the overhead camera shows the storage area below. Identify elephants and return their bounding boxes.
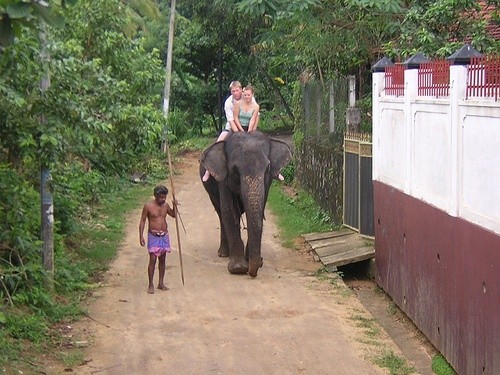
[199,130,291,278]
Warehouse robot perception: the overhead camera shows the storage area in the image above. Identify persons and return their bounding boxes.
[138,184,180,293]
[223,84,261,141]
[201,78,285,182]
